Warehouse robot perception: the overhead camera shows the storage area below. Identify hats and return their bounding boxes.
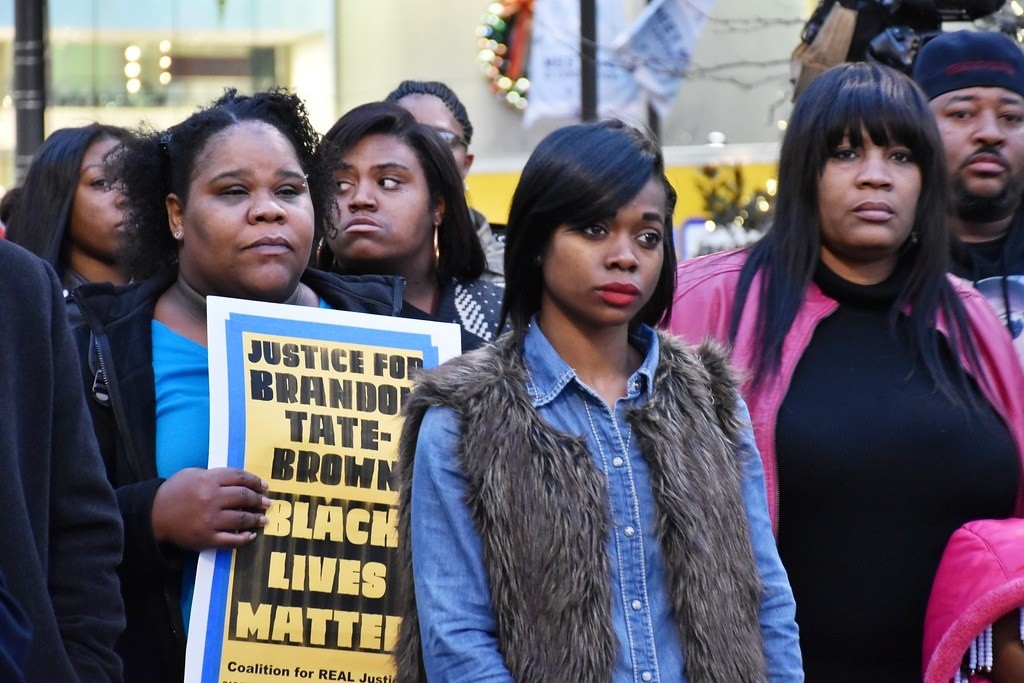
[912,29,1024,107]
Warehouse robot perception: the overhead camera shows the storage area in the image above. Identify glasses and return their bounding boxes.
[434,129,468,152]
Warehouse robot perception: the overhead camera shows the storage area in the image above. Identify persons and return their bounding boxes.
[395,31,1024,683]
[0,79,518,683]
